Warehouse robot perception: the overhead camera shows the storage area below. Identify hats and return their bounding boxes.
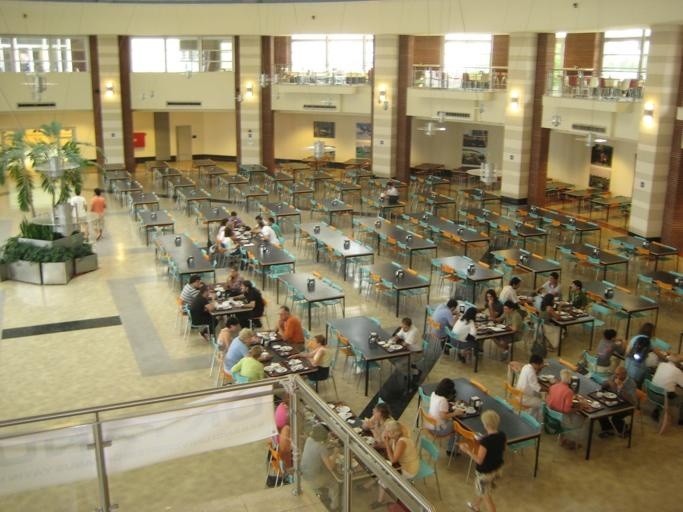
[666,351,682,363]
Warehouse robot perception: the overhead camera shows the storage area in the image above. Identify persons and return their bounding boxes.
[378,180,402,207]
[361,400,420,510]
[433,271,587,363]
[274,400,341,510]
[67,182,90,244]
[391,318,424,371]
[89,187,107,243]
[214,306,331,387]
[516,355,589,450]
[173,206,285,340]
[426,377,507,511]
[593,324,682,437]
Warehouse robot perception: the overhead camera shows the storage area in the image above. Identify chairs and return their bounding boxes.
[500,354,645,460]
[597,325,681,437]
[202,305,341,405]
[292,393,444,500]
[421,296,519,374]
[108,160,680,355]
[323,313,429,396]
[413,375,547,487]
[415,67,507,88]
[557,75,645,99]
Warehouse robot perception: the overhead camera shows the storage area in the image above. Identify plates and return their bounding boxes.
[333,427,377,470]
[232,228,252,243]
[552,299,584,320]
[538,376,619,408]
[391,345,404,350]
[303,404,353,425]
[451,405,477,415]
[255,331,306,374]
[477,320,504,333]
[214,298,245,310]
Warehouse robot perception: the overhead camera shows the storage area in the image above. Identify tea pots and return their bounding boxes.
[674,275,683,287]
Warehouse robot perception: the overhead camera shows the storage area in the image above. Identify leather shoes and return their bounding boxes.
[357,484,368,490]
[369,501,383,509]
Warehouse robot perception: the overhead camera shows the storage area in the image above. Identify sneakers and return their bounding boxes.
[200,331,210,342]
[598,430,615,440]
